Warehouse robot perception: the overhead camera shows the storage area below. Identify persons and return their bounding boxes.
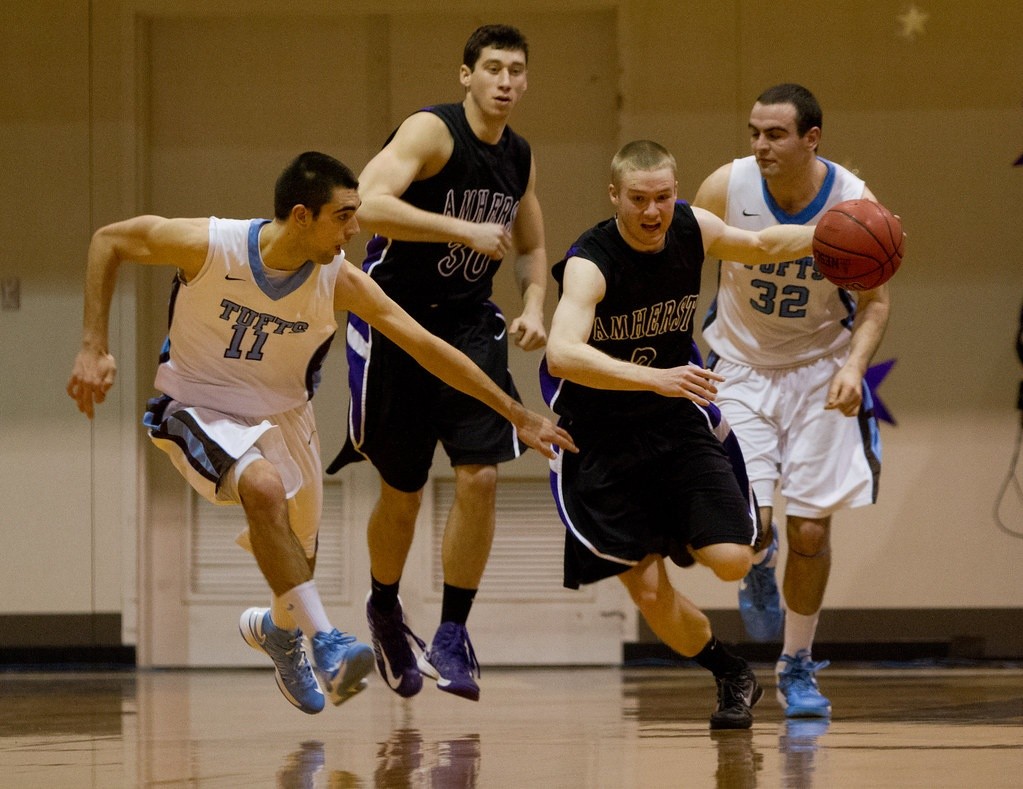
[344,26,549,700]
[66,150,578,715]
[693,83,891,716]
[539,139,907,728]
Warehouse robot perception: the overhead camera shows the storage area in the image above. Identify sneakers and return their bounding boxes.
[709,656,763,729]
[737,527,780,642]
[241,607,326,714]
[775,650,831,716]
[365,592,423,698]
[417,621,482,702]
[312,627,376,707]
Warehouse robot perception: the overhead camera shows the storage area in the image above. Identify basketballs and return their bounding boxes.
[812,198,904,291]
